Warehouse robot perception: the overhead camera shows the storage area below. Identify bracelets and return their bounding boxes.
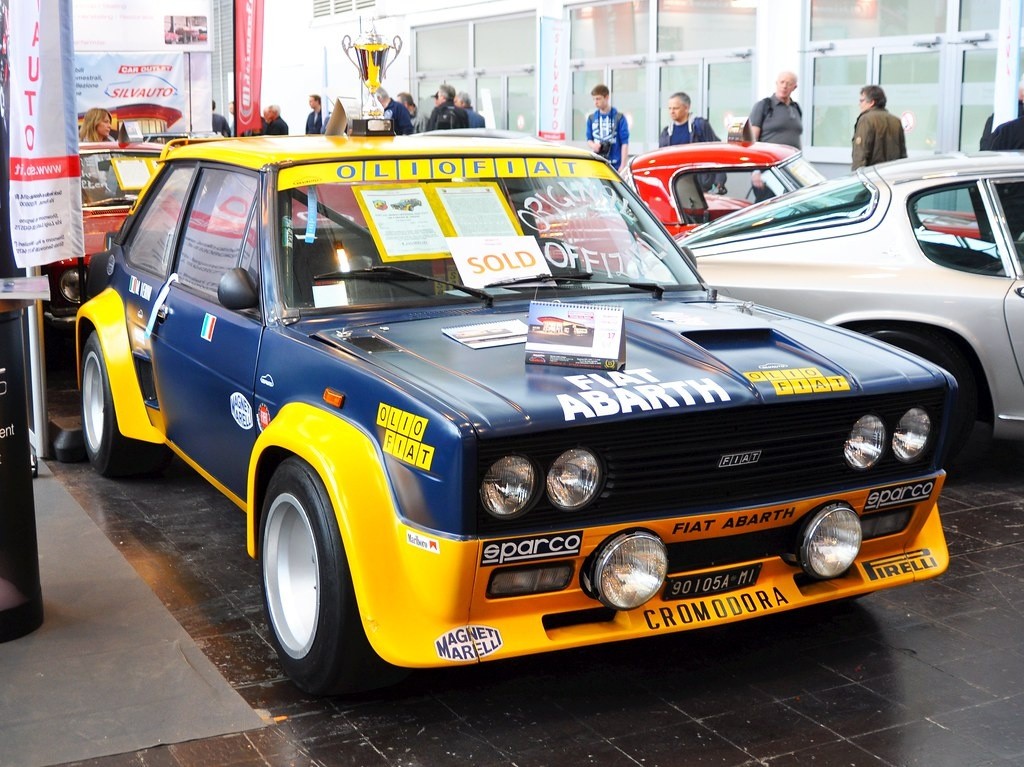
[752,170,761,174]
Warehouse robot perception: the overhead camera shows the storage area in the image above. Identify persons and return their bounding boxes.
[305,85,486,135]
[742,72,803,204]
[851,85,907,171]
[585,83,629,173]
[79,108,127,207]
[212,98,288,137]
[659,91,728,195]
[979,74,1024,240]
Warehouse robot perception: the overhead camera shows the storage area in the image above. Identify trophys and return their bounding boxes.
[342,15,403,136]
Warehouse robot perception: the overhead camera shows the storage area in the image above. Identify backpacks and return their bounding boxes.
[433,104,462,131]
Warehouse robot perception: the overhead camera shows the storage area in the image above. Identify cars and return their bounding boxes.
[623,148,1024,468]
[34,128,526,334]
[530,138,980,274]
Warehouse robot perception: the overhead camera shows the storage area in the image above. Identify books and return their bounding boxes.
[441,301,627,371]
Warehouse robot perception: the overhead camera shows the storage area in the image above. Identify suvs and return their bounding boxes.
[69,128,959,706]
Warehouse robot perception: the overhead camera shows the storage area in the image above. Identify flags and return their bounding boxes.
[9,0,86,268]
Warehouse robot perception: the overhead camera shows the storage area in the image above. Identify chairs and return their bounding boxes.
[290,228,436,308]
